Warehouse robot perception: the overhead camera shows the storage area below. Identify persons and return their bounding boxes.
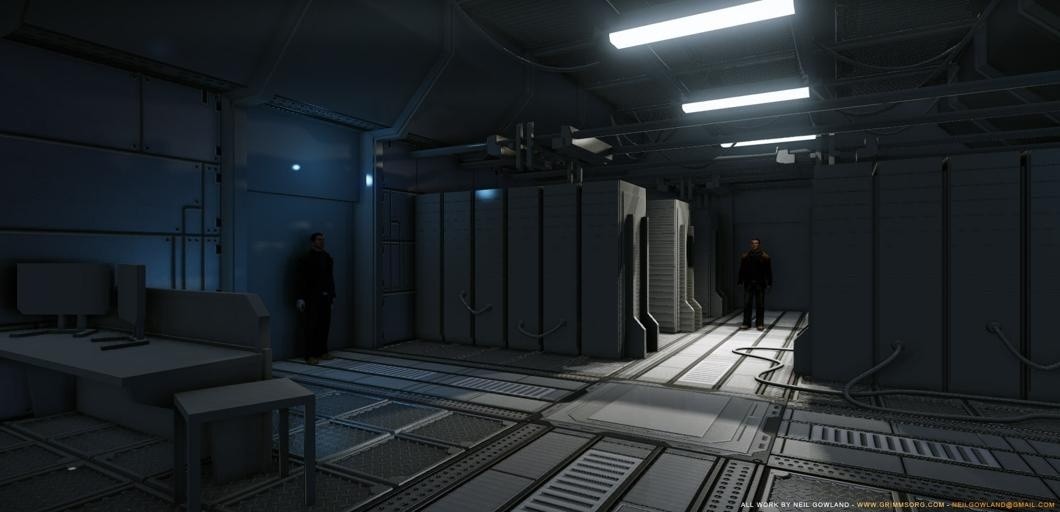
[294,230,335,363]
[737,237,774,331]
